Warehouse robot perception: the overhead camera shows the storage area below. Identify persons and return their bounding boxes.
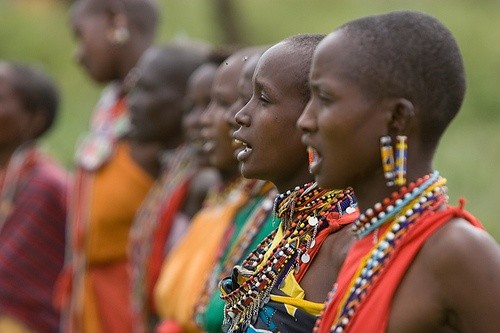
[0,0,282,333]
[217,33,349,333]
[245,9,500,333]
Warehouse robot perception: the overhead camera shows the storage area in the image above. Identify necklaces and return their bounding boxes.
[192,179,278,324]
[220,180,357,324]
[313,170,451,333]
